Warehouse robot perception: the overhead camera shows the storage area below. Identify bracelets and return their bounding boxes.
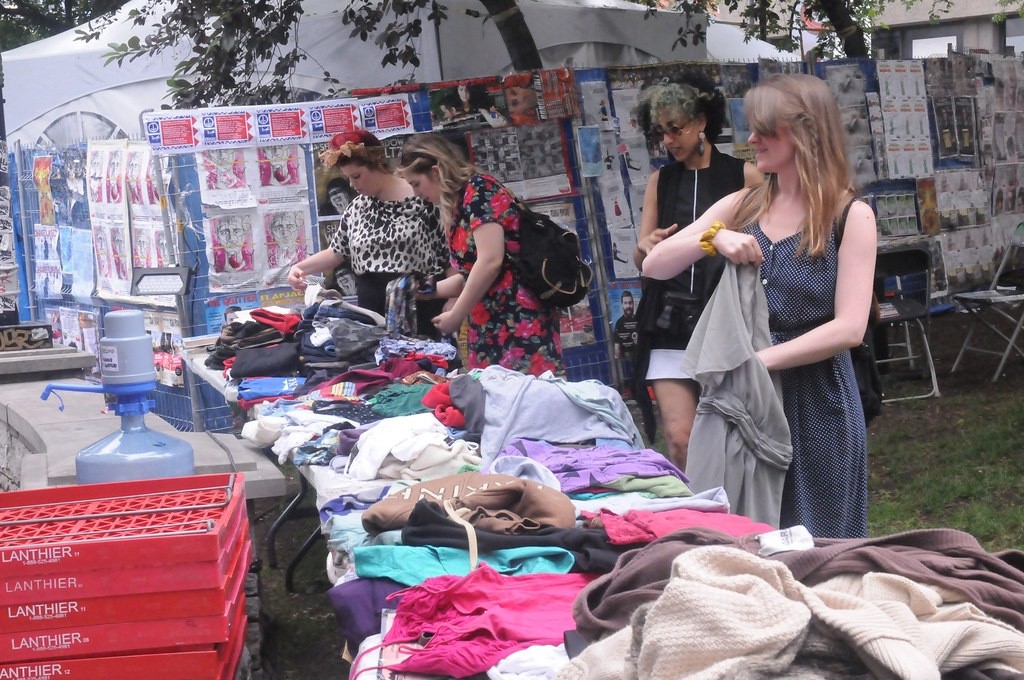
[699,221,726,258]
[636,242,646,254]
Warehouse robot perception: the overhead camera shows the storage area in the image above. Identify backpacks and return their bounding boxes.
[504,185,594,308]
[835,197,883,430]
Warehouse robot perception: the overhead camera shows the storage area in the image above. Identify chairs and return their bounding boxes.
[864,220,1024,405]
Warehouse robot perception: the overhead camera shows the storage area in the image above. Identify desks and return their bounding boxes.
[183,295,1024,680]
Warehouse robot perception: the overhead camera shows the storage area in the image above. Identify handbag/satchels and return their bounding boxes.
[655,290,701,341]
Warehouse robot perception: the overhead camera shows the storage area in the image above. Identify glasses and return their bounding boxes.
[655,115,694,141]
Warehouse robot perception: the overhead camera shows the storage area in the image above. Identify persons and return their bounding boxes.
[224,306,242,325]
[505,75,540,119]
[439,84,495,118]
[629,76,764,474]
[641,74,877,539]
[288,130,462,372]
[394,132,568,383]
[613,291,639,361]
